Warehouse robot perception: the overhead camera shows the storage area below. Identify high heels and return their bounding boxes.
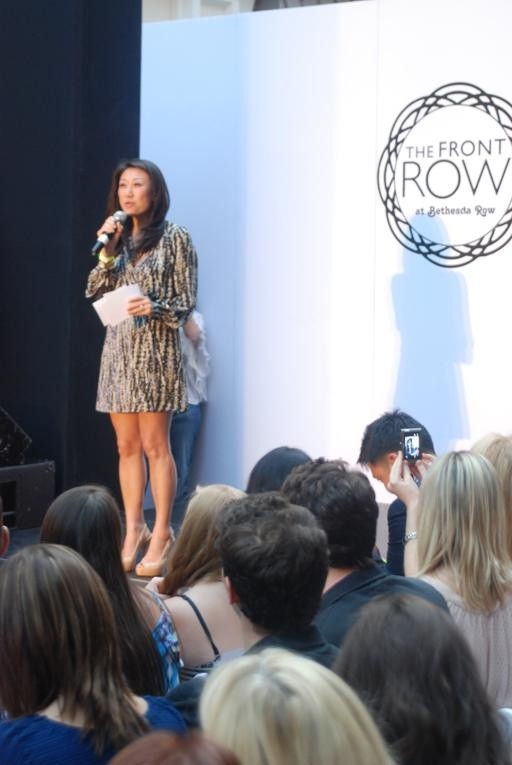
[134,526,177,577]
[120,521,153,574]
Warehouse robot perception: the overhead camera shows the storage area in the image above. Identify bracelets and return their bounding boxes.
[96,248,113,265]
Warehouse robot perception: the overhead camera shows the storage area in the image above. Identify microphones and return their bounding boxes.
[90,209,128,257]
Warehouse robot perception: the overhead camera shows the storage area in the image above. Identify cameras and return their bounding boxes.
[401,428,422,464]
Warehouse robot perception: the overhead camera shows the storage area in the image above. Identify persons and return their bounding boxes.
[169,308,205,524]
[2,413,512,764]
[86,159,198,579]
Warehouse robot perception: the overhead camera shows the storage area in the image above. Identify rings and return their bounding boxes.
[139,303,146,314]
